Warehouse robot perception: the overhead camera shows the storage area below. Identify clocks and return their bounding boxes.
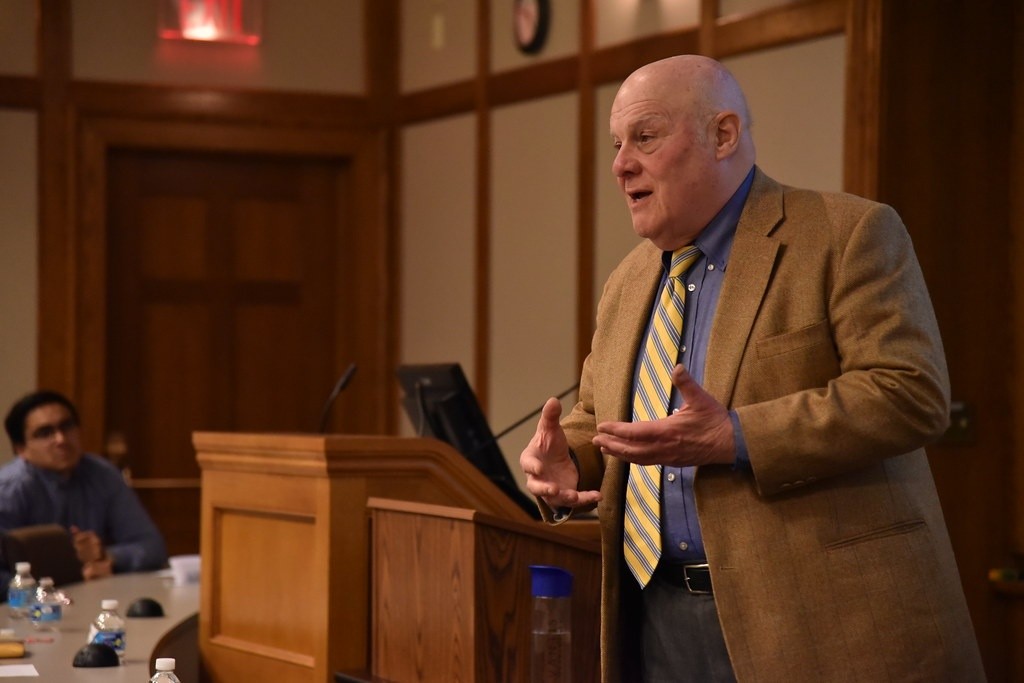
[513,0,548,55]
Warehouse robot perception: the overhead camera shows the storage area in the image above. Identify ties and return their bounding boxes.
[624,246,703,590]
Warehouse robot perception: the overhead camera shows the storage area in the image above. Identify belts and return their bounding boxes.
[620,557,713,594]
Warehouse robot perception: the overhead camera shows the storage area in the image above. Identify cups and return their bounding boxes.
[169,556,199,586]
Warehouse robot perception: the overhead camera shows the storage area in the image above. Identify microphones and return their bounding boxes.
[317,364,357,435]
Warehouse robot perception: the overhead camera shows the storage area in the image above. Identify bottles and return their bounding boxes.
[93,599,126,659]
[31,579,62,629]
[9,562,37,621]
[526,566,574,683]
[148,658,181,683]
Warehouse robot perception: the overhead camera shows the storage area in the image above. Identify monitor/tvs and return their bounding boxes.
[397,362,523,509]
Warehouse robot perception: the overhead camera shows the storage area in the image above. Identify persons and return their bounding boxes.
[0,389,168,605]
[519,55,990,683]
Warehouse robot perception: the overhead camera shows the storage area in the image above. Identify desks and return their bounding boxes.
[0,568,199,683]
